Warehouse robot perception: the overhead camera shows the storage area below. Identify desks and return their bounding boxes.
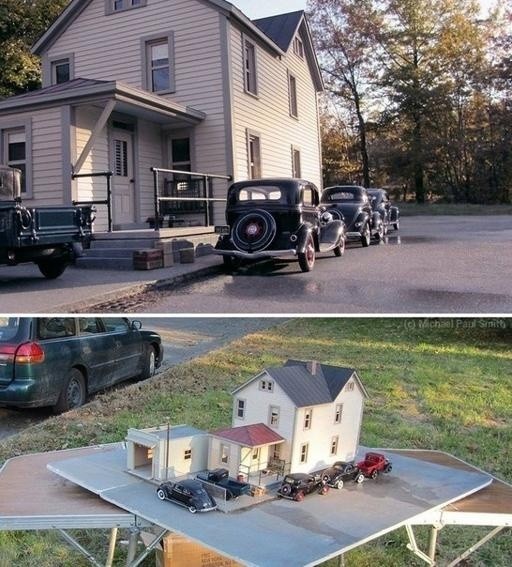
[0,439,512,567]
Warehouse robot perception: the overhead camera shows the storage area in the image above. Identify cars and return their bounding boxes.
[156,478,218,515]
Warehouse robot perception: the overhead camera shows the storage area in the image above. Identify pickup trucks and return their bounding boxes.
[198,468,252,502]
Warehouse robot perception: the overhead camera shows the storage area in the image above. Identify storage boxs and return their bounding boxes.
[137,524,245,567]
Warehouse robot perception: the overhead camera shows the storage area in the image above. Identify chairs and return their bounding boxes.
[39,318,49,337]
[56,318,89,336]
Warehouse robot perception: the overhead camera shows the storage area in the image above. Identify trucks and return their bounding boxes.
[0,164,98,280]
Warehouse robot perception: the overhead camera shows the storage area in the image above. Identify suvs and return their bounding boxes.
[278,452,393,501]
[1,318,166,414]
[214,176,402,271]
